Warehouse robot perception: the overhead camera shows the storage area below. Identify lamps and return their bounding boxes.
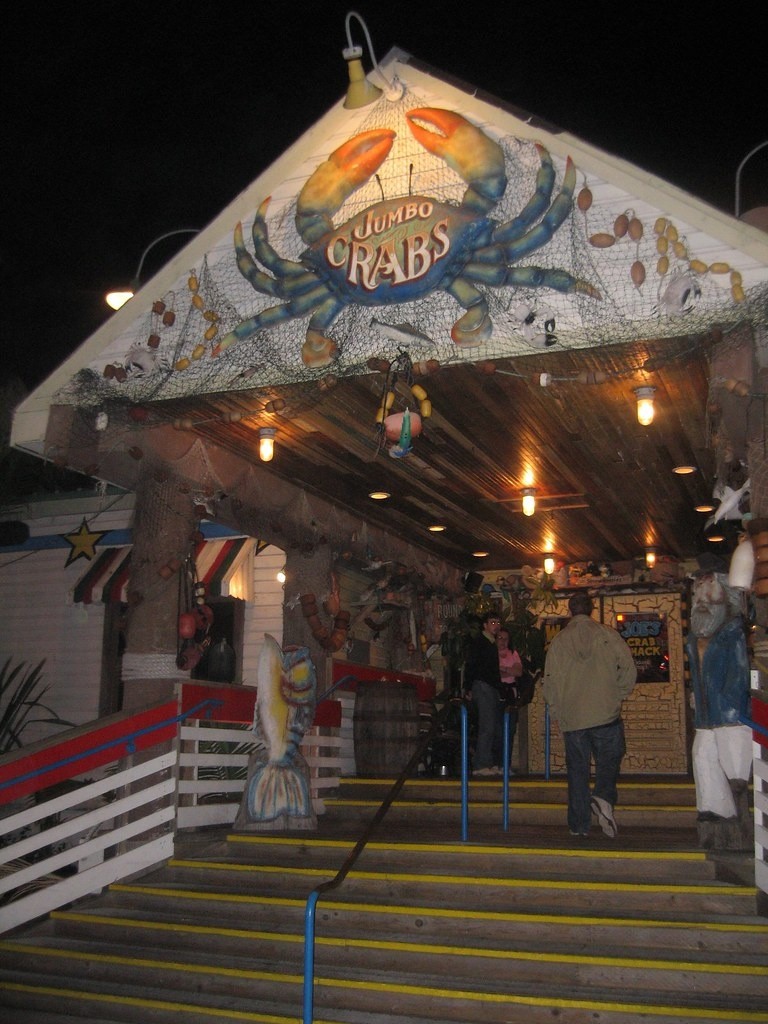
[106,228,202,311]
[460,572,484,593]
[342,11,405,108]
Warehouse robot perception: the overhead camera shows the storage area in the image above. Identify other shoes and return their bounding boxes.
[472,764,515,775]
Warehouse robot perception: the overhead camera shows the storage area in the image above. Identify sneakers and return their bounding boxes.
[568,827,589,836]
[589,796,618,838]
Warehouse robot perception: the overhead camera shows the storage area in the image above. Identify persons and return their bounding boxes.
[465,613,523,776]
[687,550,755,819]
[542,593,637,837]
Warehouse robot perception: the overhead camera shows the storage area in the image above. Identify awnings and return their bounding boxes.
[66,536,254,605]
